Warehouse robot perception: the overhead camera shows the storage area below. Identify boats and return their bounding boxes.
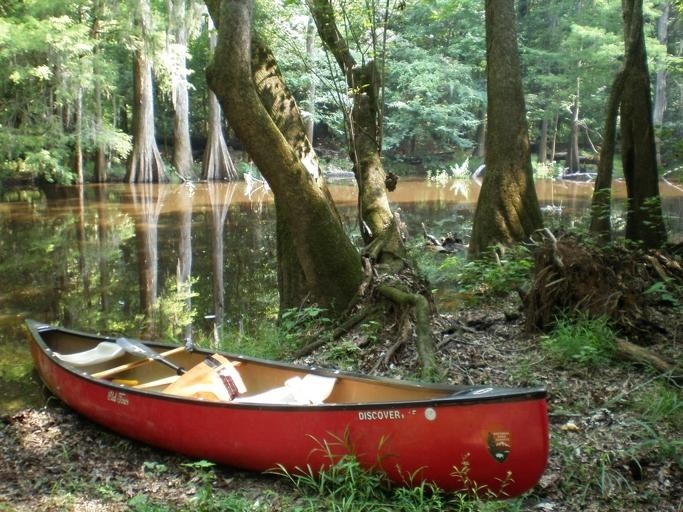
[18,317,548,501]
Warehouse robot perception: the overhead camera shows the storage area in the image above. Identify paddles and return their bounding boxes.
[116,337,186,375]
